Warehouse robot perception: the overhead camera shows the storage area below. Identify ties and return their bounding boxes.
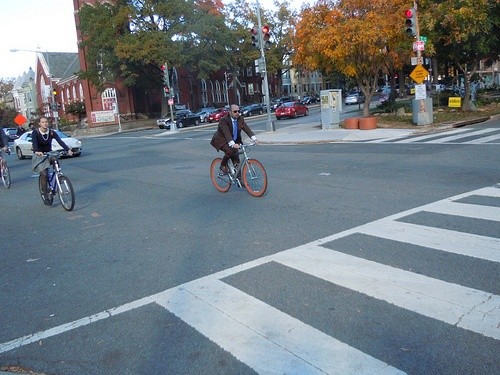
[232,121,237,140]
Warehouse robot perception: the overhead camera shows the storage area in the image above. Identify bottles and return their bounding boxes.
[229,164,235,174]
[50,173,54,187]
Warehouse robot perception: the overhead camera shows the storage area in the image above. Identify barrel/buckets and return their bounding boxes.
[344,119,359,129]
[359,116,376,130]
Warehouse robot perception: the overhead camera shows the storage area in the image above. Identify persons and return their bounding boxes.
[210,104,260,188]
[32,117,73,206]
[0,128,9,152]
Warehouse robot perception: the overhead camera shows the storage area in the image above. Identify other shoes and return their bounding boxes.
[238,182,248,187]
[220,164,228,173]
[41,194,52,206]
[58,177,64,184]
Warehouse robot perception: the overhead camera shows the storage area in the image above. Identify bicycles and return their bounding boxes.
[0,149,11,188]
[210,142,268,197]
[37,151,75,211]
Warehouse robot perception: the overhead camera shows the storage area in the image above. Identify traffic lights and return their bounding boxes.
[405,8,418,37]
[52,89,56,99]
[161,64,170,93]
[251,28,261,49]
[262,26,271,47]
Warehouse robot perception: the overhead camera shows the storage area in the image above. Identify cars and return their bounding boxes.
[156,110,201,130]
[195,108,229,123]
[345,92,365,105]
[4,128,18,142]
[276,101,309,120]
[14,129,82,160]
[241,97,317,117]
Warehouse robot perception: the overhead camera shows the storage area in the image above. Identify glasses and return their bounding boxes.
[233,110,240,113]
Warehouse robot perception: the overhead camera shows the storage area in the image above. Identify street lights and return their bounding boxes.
[10,49,59,129]
